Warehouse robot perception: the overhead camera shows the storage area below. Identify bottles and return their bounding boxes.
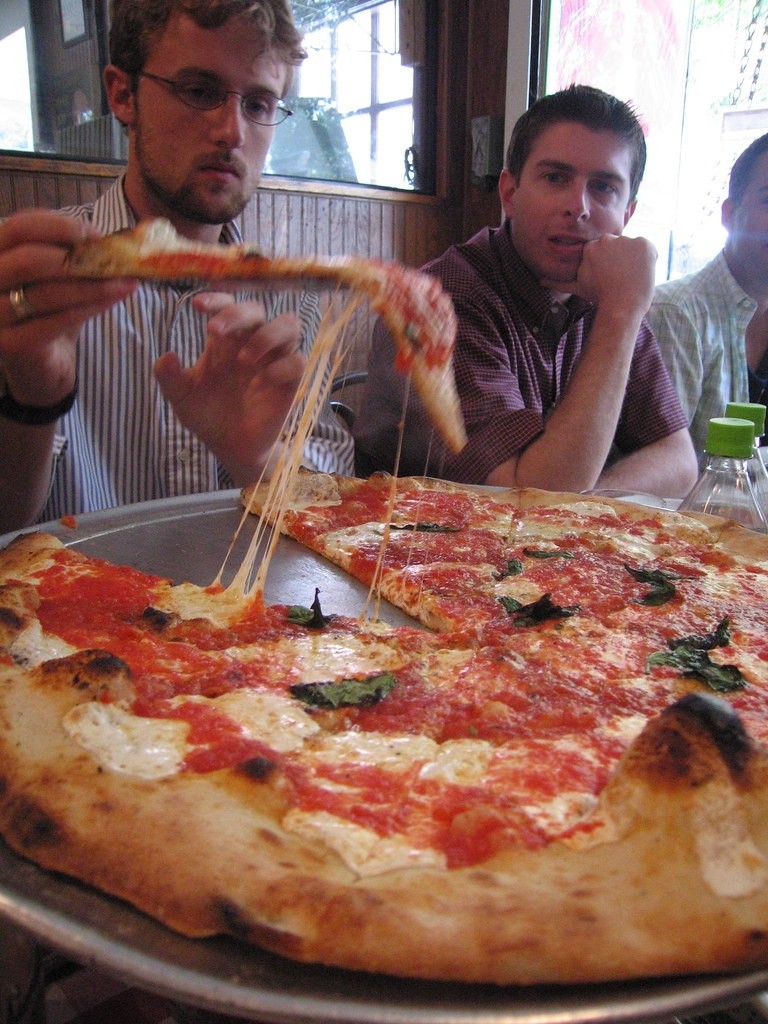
[722,403,768,527]
[676,416,762,533]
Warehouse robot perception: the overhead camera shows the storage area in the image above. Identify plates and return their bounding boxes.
[613,493,687,513]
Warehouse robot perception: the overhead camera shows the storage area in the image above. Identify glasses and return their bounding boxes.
[110,58,295,127]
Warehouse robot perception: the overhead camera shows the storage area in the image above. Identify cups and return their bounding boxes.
[572,487,666,513]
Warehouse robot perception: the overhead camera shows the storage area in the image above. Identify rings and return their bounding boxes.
[8,286,35,320]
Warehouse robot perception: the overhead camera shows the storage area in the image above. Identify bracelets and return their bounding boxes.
[0,376,84,424]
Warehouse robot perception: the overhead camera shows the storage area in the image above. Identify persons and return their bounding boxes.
[0,0,357,536]
[642,135,768,483]
[358,87,702,497]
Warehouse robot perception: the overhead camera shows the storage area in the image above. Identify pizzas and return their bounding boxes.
[58,213,467,457]
[2,471,768,985]
